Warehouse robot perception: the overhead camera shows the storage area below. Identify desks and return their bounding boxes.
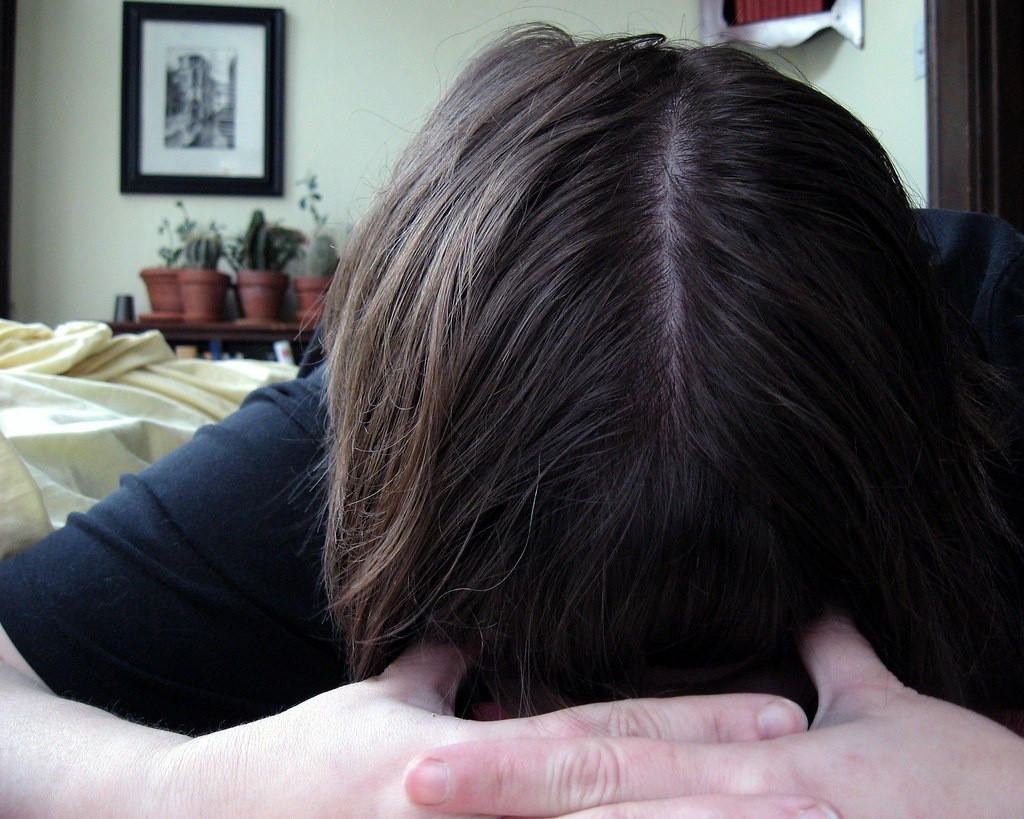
[105,322,311,356]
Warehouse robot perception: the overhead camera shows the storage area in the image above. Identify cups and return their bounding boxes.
[114,296,135,323]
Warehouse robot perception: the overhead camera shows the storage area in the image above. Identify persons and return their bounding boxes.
[0,26,1023,819]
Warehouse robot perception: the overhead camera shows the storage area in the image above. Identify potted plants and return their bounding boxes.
[140,176,341,327]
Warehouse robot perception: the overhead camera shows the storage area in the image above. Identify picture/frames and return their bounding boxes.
[120,0,285,197]
